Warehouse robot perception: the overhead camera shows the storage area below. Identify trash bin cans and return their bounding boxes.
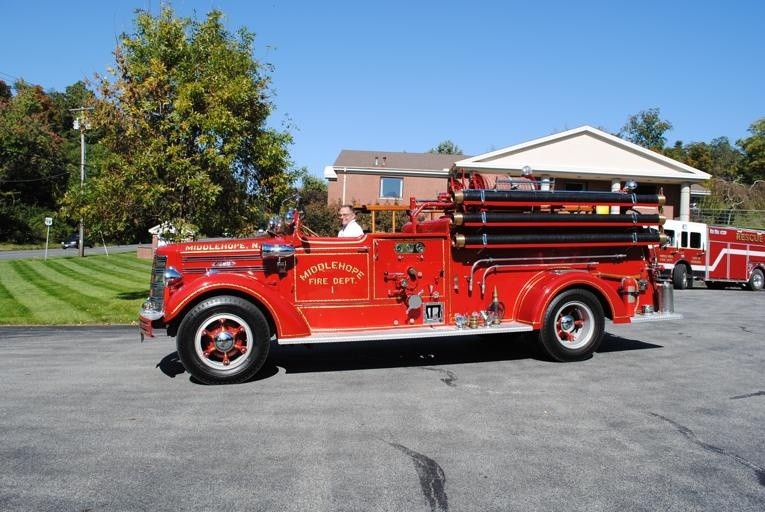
[657,283,674,314]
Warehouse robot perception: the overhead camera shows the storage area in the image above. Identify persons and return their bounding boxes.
[337,206,364,238]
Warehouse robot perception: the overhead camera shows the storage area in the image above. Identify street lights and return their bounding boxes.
[65,104,98,260]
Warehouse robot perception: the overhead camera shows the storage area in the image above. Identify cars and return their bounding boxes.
[61,230,94,251]
[221,229,232,238]
[258,228,264,233]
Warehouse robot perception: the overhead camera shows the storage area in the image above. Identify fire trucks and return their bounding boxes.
[644,218,765,292]
[137,164,682,383]
